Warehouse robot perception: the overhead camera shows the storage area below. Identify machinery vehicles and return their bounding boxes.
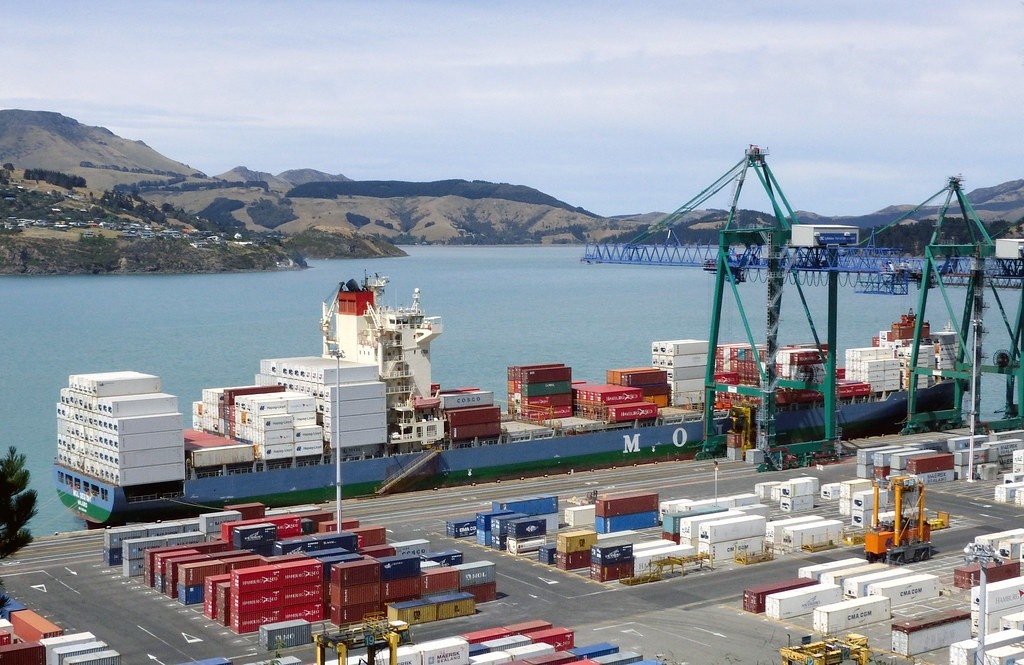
[863,476,933,564]
